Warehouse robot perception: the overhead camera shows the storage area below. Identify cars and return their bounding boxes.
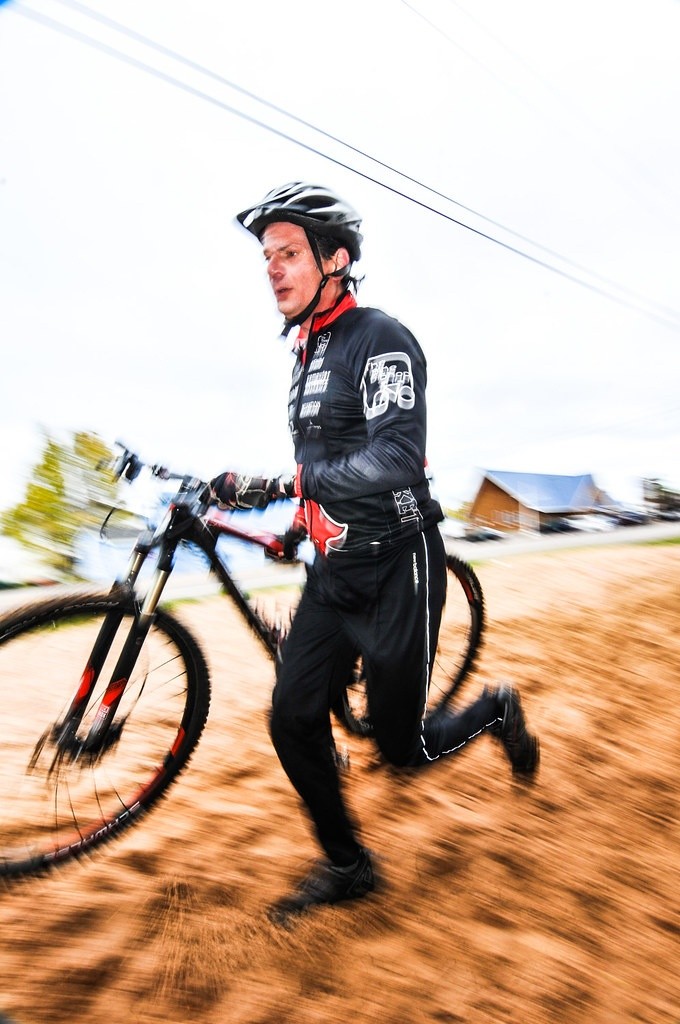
[433,504,680,538]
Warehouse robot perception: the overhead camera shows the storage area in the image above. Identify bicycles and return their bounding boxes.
[0,442,492,882]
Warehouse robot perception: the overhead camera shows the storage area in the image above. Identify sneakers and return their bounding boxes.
[268,847,376,925]
[487,686,539,777]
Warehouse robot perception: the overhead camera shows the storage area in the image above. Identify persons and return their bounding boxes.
[209,182,541,913]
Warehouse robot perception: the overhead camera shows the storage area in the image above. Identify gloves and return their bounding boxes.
[199,471,277,512]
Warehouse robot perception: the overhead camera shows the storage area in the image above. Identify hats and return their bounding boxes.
[237,181,363,247]
